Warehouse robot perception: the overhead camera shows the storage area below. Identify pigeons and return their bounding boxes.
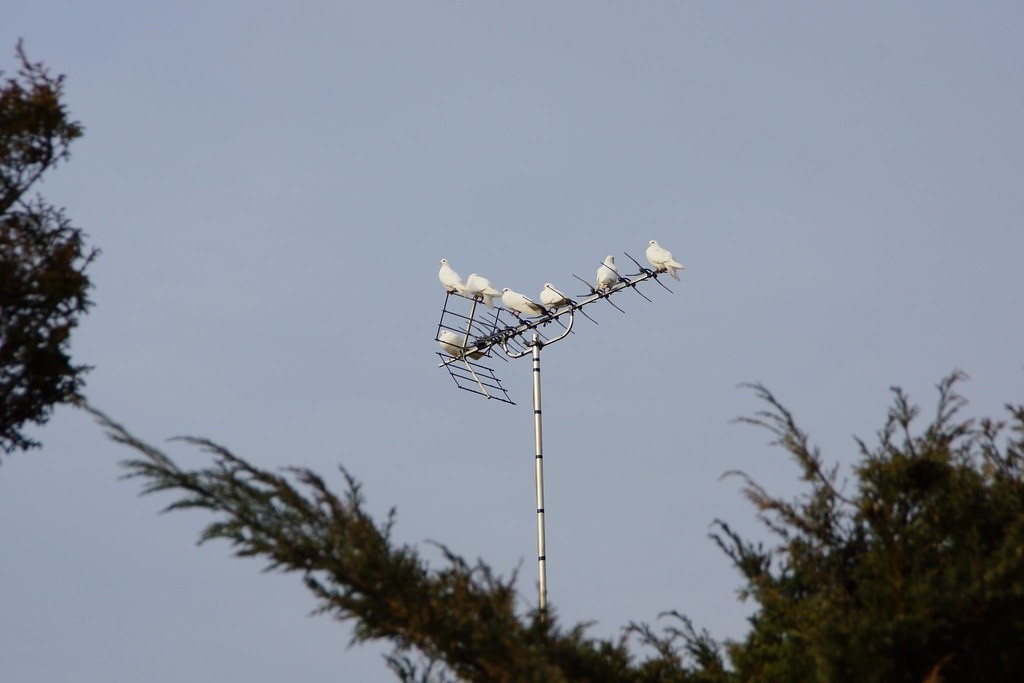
[438,239,685,357]
